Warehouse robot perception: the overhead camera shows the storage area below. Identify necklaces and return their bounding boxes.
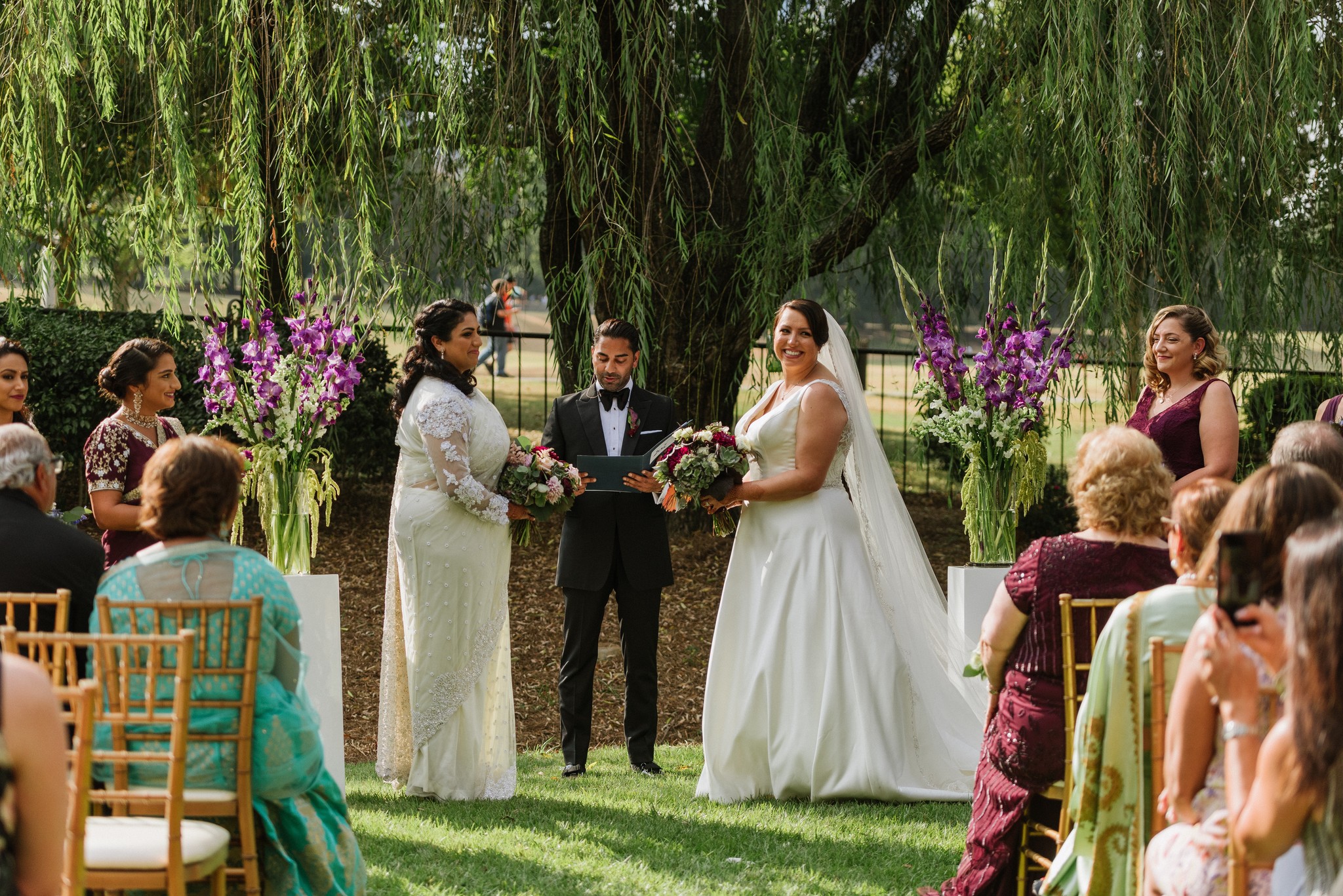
[1158,376,1195,403]
[1178,573,1217,582]
[120,403,161,429]
[781,360,818,399]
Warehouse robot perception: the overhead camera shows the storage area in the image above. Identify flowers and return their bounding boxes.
[188,215,392,574]
[498,435,582,547]
[888,217,1094,563]
[626,406,640,437]
[652,421,764,537]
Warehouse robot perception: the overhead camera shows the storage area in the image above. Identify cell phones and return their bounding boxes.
[1218,530,1267,627]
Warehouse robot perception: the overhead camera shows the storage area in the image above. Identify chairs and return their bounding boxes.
[1017,594,1125,896]
[0,589,261,896]
[1151,638,1182,833]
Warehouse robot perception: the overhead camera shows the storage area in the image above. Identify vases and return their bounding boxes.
[973,468,1016,568]
[271,471,310,575]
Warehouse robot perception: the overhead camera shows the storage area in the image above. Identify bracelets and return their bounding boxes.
[1224,721,1258,740]
[986,686,999,694]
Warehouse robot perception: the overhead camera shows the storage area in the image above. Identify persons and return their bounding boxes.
[83,336,194,572]
[0,422,104,750]
[0,652,72,896]
[541,319,694,777]
[0,336,38,434]
[694,298,989,804]
[76,434,370,896]
[1041,390,1343,896]
[1124,304,1240,495]
[483,276,521,376]
[1197,504,1342,896]
[918,424,1175,896]
[475,277,515,376]
[377,299,534,798]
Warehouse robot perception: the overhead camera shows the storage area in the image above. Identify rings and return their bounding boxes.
[528,510,531,513]
[705,500,710,506]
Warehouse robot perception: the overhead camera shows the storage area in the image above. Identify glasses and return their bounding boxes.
[48,455,65,473]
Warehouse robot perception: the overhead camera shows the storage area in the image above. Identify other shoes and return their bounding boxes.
[561,764,585,778]
[631,762,663,777]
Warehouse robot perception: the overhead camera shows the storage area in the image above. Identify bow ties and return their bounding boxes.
[598,387,630,412]
